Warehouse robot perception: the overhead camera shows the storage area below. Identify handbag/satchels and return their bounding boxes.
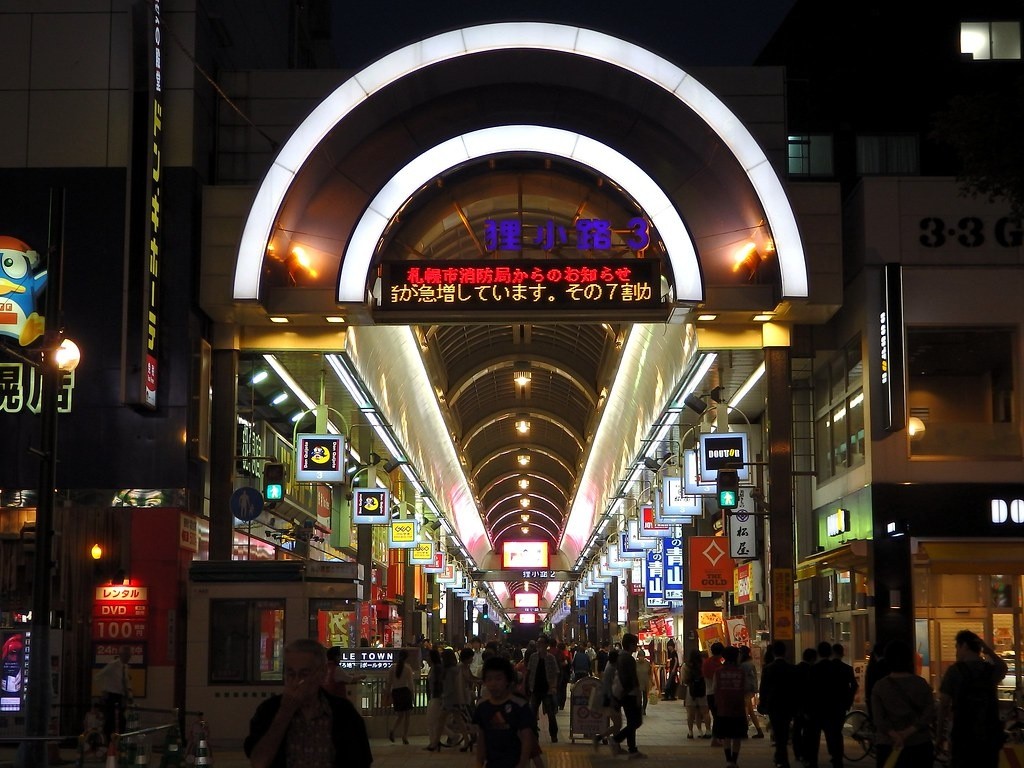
[587,684,615,717]
[612,669,624,700]
[381,691,393,707]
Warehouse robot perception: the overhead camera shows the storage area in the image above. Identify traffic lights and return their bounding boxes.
[263,462,285,503]
[716,468,739,510]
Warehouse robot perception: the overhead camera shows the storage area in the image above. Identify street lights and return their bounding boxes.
[22,187,82,767]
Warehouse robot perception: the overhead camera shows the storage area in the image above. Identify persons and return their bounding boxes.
[244,640,373,768]
[96,646,131,743]
[422,647,460,752]
[816,641,843,767]
[325,646,366,696]
[783,646,820,768]
[663,640,679,701]
[681,649,712,739]
[594,648,628,755]
[427,649,470,754]
[759,641,795,767]
[609,632,649,760]
[869,641,937,767]
[701,641,725,746]
[368,631,621,711]
[737,645,765,738]
[471,657,530,768]
[714,646,747,768]
[831,645,858,761]
[633,650,652,715]
[453,647,479,742]
[936,632,1008,768]
[382,650,410,743]
[525,637,559,743]
[695,650,712,738]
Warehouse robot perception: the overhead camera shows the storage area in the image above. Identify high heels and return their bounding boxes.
[427,741,441,753]
[402,736,410,745]
[460,741,474,752]
[388,733,395,743]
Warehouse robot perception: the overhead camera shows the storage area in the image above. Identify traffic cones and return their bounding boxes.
[106,712,214,767]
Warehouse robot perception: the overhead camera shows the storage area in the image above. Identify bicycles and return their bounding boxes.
[841,708,953,765]
[439,682,481,747]
[1001,688,1024,745]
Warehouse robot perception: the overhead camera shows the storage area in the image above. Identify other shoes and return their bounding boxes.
[608,735,619,758]
[699,733,723,748]
[741,734,749,740]
[591,733,600,754]
[617,746,628,755]
[551,734,558,743]
[687,732,694,739]
[724,760,739,768]
[627,751,648,760]
[773,757,819,768]
[751,730,764,739]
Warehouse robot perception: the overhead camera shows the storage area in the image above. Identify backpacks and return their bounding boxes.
[685,661,707,701]
